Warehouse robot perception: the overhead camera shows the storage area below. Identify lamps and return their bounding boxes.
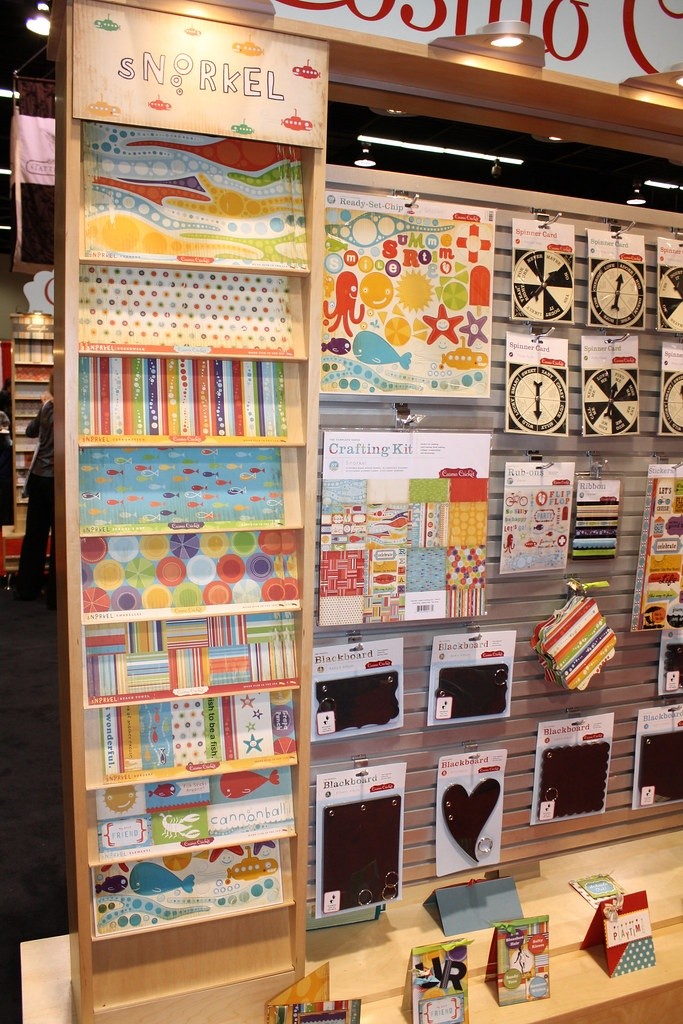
[619,62,683,99]
[25,3,52,36]
[429,20,546,68]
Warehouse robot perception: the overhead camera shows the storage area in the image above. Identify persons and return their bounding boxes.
[15,369,57,610]
[0,378,14,526]
